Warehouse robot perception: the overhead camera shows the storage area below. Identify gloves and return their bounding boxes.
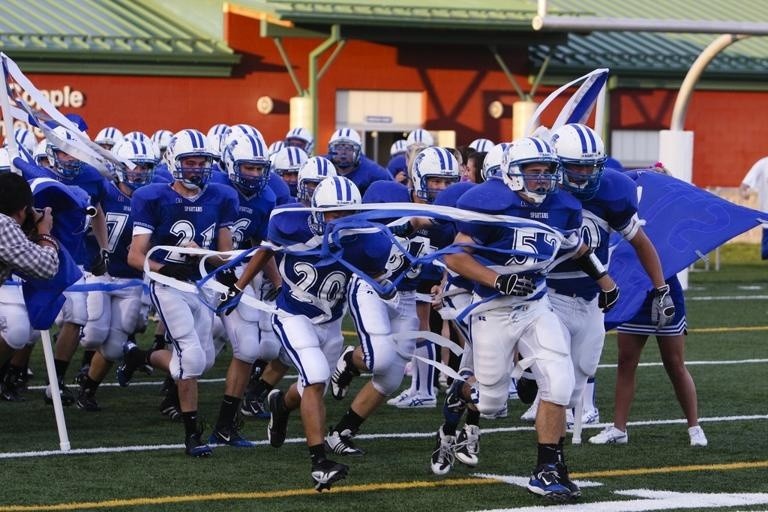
[214,283,242,316]
[597,283,621,314]
[157,261,194,282]
[493,272,537,296]
[90,248,110,276]
[652,284,676,331]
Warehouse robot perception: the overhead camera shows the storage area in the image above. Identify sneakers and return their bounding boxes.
[480,398,508,419]
[521,399,540,422]
[1,356,100,411]
[310,458,350,492]
[267,389,286,448]
[208,423,254,447]
[429,367,479,475]
[240,359,271,418]
[324,427,366,458]
[116,340,180,421]
[528,461,583,501]
[687,426,707,447]
[184,432,214,458]
[564,410,629,446]
[387,362,448,410]
[331,343,362,400]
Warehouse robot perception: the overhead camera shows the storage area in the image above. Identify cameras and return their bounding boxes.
[29,209,43,228]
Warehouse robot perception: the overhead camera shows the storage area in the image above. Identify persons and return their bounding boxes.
[739,155,768,260]
[2,123,709,498]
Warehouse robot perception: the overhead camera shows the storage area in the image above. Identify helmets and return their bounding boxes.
[3,123,271,195]
[391,128,462,202]
[468,122,607,205]
[267,127,362,214]
